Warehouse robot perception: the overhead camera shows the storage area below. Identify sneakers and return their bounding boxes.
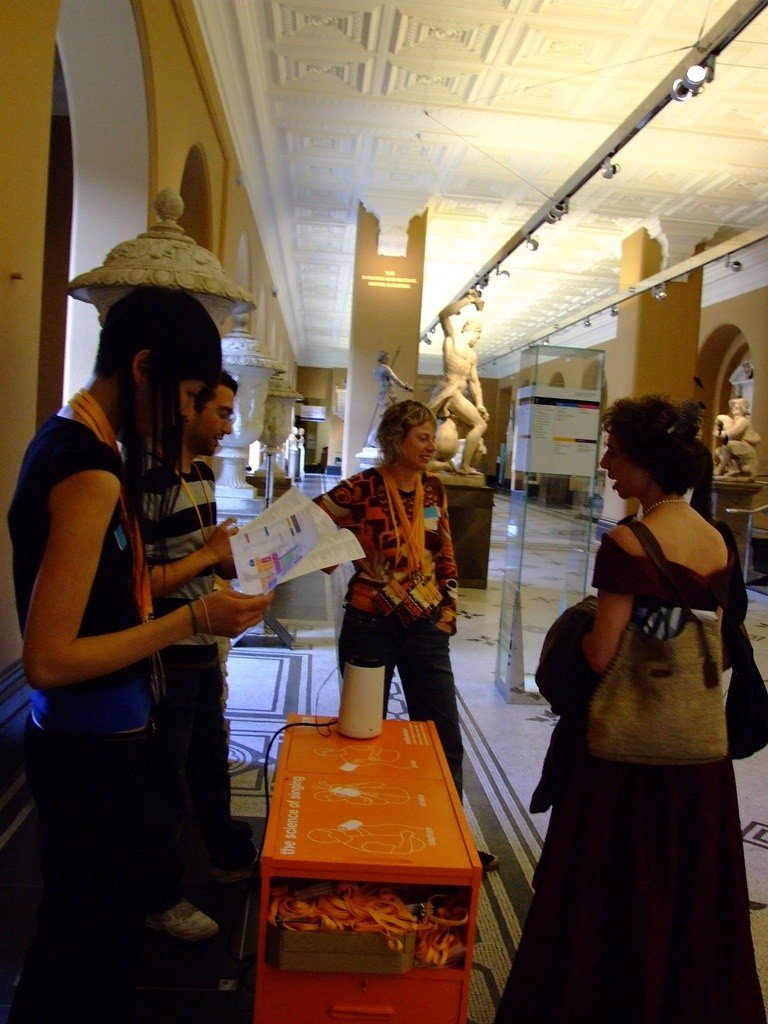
[214,849,260,884]
[145,899,219,941]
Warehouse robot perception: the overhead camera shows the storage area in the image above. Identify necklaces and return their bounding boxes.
[158,448,213,544]
[643,499,690,517]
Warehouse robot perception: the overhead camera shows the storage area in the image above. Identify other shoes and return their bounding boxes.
[478,850,499,871]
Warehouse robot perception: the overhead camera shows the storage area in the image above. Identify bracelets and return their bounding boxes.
[199,597,213,636]
[187,602,197,637]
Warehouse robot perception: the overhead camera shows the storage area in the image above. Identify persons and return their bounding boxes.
[311,399,499,874]
[714,398,761,481]
[494,396,768,1023]
[6,288,273,1024]
[368,349,415,448]
[426,292,491,476]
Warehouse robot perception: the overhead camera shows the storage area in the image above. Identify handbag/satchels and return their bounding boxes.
[586,604,728,768]
